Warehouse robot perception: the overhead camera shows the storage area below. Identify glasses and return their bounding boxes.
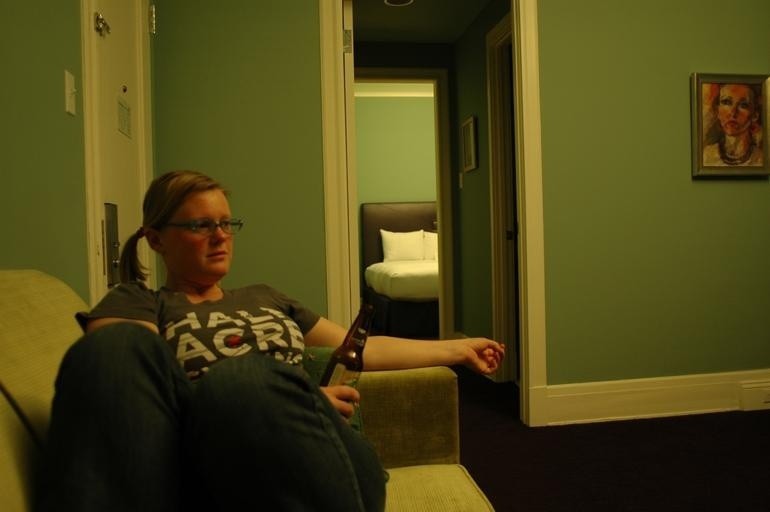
[165,218,243,234]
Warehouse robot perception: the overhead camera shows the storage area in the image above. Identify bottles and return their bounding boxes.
[318,303,376,420]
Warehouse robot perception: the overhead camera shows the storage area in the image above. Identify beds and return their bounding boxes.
[361,202,440,340]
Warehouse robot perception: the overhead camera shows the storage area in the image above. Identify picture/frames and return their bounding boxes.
[689,71,770,181]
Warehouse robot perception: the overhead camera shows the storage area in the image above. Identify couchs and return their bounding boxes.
[1,267,497,512]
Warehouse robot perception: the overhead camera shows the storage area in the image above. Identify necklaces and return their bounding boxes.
[718,136,754,165]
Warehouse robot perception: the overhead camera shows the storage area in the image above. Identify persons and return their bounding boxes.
[44,170,509,512]
[701,82,763,167]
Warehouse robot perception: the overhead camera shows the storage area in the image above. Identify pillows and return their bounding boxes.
[379,228,439,263]
